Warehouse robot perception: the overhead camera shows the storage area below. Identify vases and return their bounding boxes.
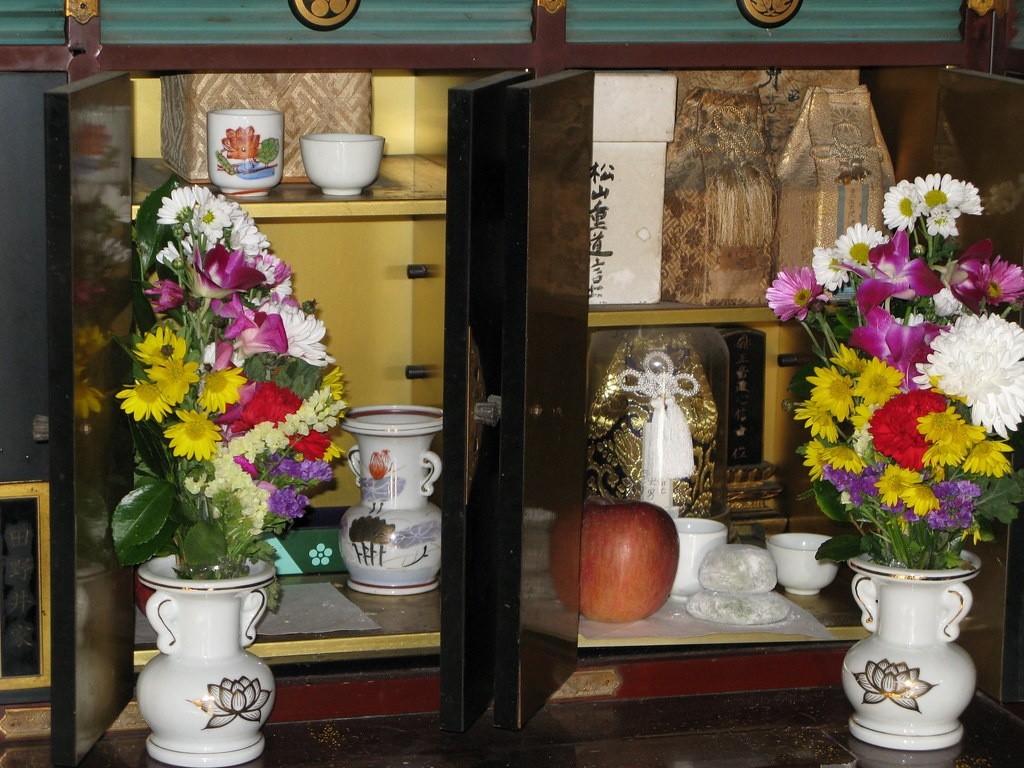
[339,405,444,585]
[135,554,276,768]
[843,548,982,751]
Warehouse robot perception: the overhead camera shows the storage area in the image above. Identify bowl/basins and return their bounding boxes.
[299,133,385,197]
[765,532,840,596]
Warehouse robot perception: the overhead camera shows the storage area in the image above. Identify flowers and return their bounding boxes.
[767,174,1024,572]
[111,174,351,611]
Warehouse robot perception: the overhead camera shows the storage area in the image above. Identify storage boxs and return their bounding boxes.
[588,141,667,305]
[593,69,678,143]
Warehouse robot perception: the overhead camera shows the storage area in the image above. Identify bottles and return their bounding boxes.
[337,405,444,596]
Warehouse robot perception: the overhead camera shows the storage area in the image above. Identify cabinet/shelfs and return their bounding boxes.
[0,1,1024,767]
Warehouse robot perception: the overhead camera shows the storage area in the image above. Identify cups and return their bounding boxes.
[207,108,284,197]
[671,518,728,601]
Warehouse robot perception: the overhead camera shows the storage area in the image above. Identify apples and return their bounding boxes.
[549,496,679,621]
